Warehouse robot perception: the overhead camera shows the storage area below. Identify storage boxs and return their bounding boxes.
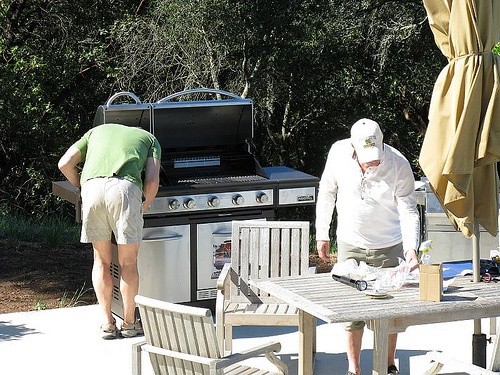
[419,262,443,302]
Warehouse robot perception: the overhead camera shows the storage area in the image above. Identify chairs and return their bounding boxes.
[216,220,316,358]
[425,326,500,375]
[132,295,290,375]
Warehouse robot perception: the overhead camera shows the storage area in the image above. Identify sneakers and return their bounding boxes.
[100,317,116,339]
[121,317,144,338]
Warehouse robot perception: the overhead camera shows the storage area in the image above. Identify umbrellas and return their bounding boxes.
[418,1,500,336]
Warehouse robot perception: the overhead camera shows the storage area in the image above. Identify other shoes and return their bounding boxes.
[388,365,398,375]
[345,371,356,375]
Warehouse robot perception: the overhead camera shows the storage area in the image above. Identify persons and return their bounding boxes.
[315,118,421,375]
[57,119,162,341]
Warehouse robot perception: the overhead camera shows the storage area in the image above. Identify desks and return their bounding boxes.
[247,266,500,375]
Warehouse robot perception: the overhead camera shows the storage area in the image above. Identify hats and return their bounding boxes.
[350,118,383,163]
